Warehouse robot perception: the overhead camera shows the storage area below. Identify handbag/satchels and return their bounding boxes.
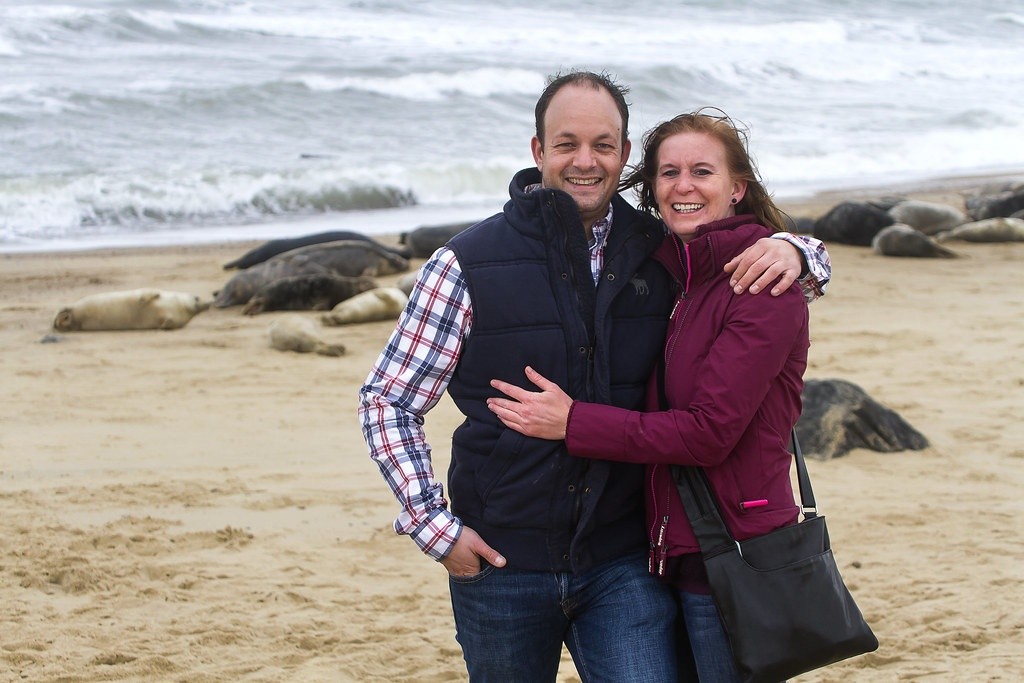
[702,515,879,683]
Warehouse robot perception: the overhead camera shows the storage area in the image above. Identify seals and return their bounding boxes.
[52,220,485,357]
[781,181,1023,461]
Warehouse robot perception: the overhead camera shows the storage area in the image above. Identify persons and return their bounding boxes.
[487,107,810,683]
[358,72,831,683]
